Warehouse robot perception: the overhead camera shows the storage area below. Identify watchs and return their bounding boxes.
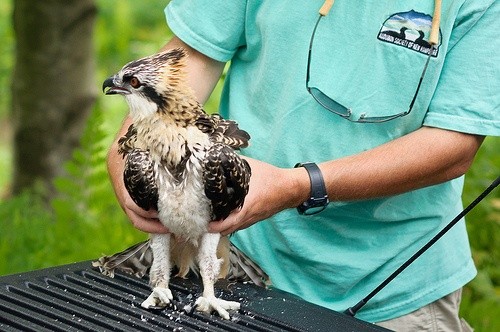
[294,161,329,216]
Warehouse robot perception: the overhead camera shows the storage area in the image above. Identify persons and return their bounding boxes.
[104,0,500,332]
